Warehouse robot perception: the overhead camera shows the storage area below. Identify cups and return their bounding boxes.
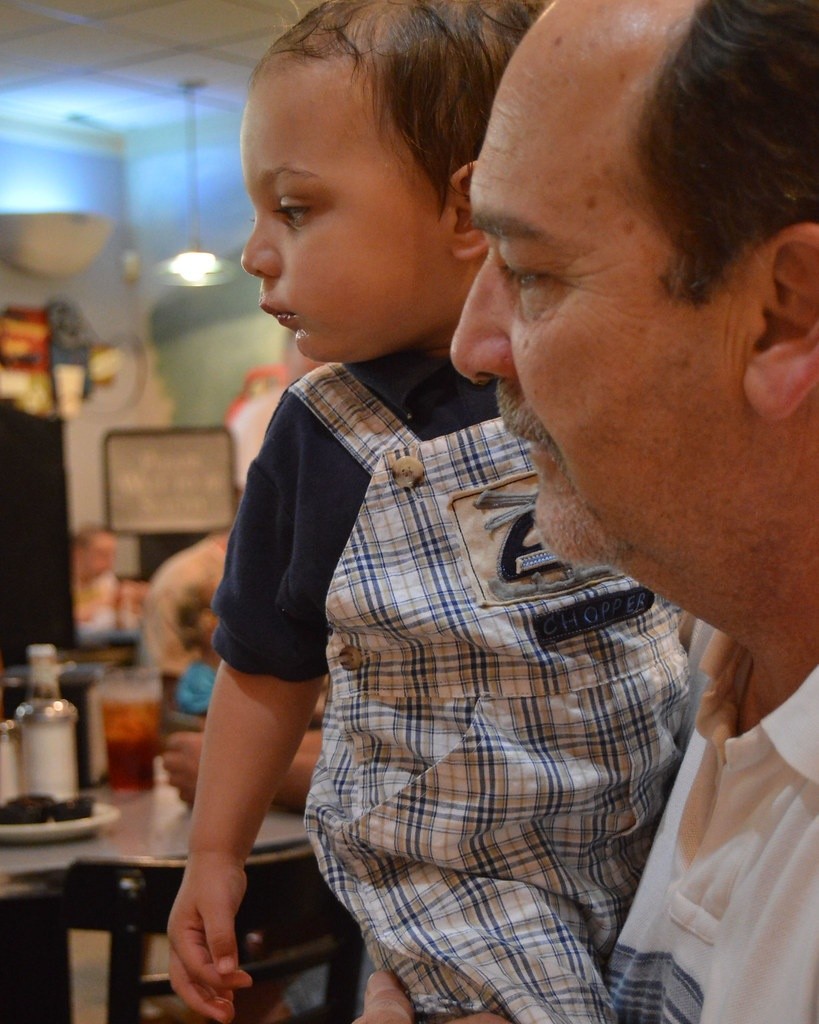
[99,665,164,796]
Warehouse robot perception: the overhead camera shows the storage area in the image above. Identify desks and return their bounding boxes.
[0,756,308,1024]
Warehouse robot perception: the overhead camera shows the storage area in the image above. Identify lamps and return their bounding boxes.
[1,213,115,275]
[154,82,234,289]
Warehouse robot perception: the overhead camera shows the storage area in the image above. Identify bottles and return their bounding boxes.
[19,644,79,822]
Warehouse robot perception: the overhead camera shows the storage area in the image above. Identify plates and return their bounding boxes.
[0,802,122,844]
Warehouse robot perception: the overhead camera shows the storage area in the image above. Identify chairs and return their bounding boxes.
[66,853,362,1024]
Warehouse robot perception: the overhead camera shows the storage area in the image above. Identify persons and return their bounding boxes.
[169,0,698,1024]
[352,0,819,1024]
[73,526,126,619]
[146,526,236,711]
[164,699,376,1024]
